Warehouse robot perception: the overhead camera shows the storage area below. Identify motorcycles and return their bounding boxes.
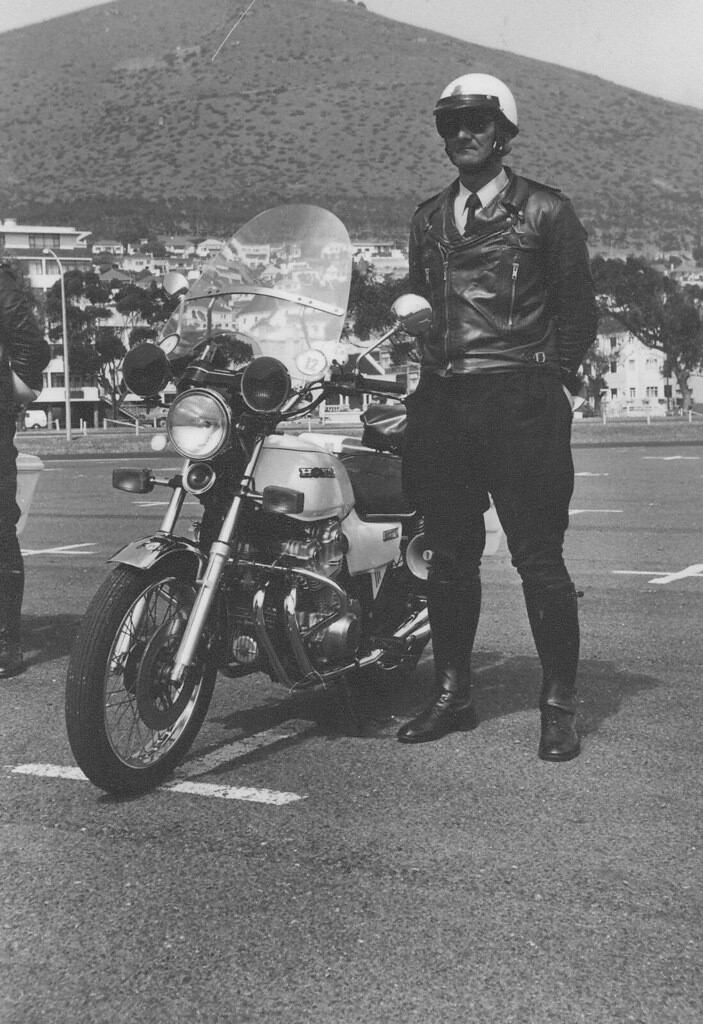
[65,203,492,796]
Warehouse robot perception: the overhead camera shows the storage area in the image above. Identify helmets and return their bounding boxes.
[432,72,519,142]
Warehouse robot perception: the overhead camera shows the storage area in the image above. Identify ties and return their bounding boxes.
[465,193,482,232]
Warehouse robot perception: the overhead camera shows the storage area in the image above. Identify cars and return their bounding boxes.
[144,403,170,428]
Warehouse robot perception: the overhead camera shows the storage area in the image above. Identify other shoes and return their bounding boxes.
[0,653,26,680]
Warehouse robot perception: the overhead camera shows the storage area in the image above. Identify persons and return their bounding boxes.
[399,72,598,761]
[0,257,50,678]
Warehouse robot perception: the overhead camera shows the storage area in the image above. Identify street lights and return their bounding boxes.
[43,246,72,443]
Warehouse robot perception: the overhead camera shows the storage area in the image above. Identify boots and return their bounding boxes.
[523,581,579,763]
[396,580,481,742]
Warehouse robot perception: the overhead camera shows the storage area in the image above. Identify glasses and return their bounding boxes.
[437,113,495,138]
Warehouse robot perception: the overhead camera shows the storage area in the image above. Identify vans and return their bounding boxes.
[22,409,48,430]
